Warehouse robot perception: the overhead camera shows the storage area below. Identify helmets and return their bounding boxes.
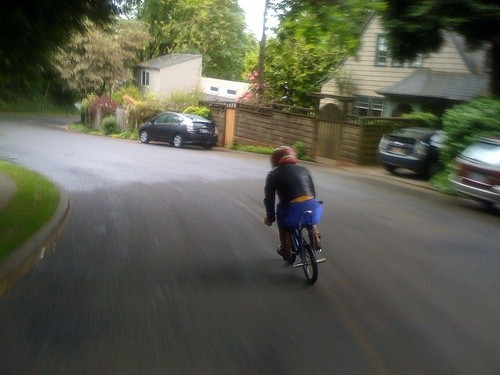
[271,145,297,168]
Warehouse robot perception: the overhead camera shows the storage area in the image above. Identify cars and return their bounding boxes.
[138,112,218,152]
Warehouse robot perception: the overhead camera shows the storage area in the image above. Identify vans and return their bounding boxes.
[447,137,500,214]
[378,126,444,182]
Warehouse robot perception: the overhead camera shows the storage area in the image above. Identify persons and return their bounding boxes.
[264,146,323,257]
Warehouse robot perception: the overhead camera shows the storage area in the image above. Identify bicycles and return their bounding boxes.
[265,200,327,284]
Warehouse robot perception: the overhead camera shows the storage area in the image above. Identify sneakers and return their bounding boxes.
[308,230,321,250]
[277,244,297,265]
